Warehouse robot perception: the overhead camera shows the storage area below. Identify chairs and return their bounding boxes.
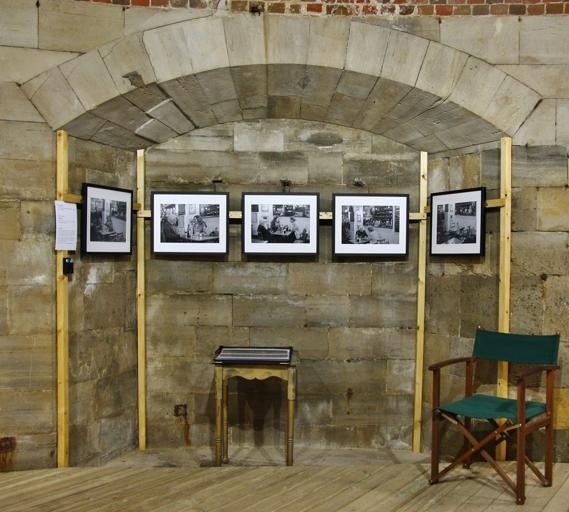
[427,328,562,507]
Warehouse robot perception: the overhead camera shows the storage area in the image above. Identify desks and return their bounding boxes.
[209,350,299,467]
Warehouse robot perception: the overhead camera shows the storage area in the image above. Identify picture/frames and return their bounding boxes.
[212,345,293,367]
[428,186,488,258]
[149,191,410,259]
[80,181,135,258]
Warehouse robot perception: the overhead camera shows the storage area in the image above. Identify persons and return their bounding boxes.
[163,215,187,241]
[288,216,303,239]
[189,215,209,236]
[354,225,367,242]
[258,217,271,240]
[271,215,281,230]
[368,226,379,243]
